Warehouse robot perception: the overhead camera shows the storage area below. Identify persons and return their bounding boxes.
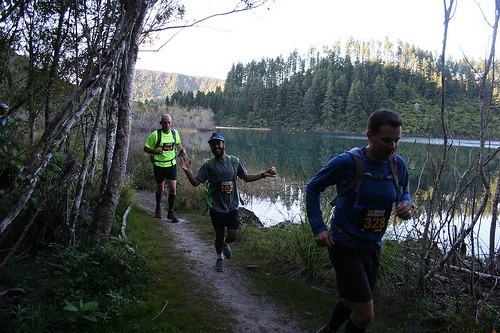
[0,103,12,138]
[180,133,276,270]
[144,114,187,223]
[306,110,414,333]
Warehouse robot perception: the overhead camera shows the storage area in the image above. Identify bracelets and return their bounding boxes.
[262,173,265,178]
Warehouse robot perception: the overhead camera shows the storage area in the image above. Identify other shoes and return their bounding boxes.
[315,324,332,333]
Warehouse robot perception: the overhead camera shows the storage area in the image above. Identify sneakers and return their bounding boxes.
[222,242,233,259]
[215,257,224,271]
[155,205,162,217]
[167,211,179,223]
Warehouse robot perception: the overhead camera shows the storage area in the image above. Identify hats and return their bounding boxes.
[208,133,225,143]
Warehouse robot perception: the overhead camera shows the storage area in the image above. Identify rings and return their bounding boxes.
[409,211,411,214]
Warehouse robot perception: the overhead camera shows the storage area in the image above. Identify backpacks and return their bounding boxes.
[201,156,239,209]
